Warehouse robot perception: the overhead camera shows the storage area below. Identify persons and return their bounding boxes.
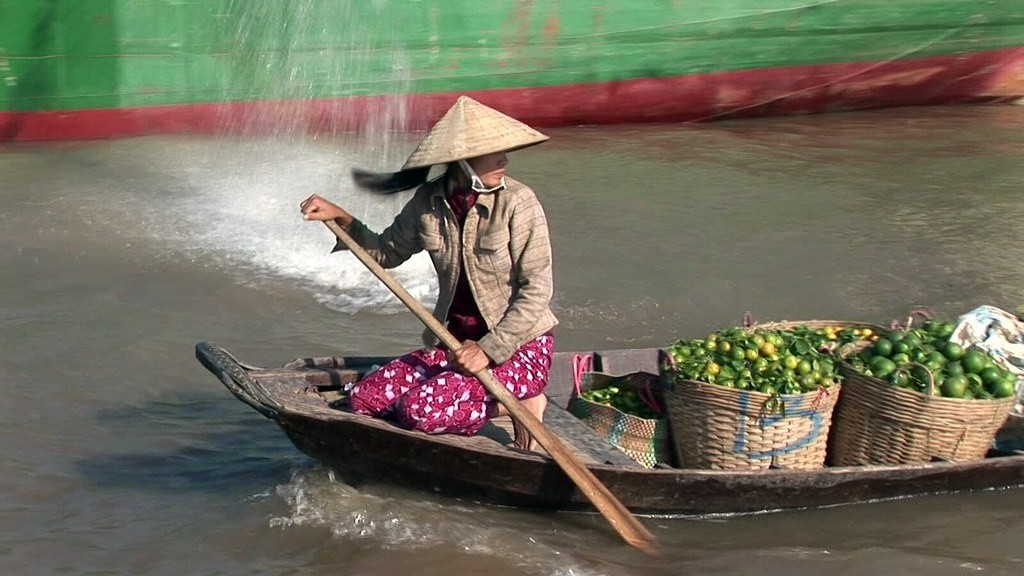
[300,95,559,452]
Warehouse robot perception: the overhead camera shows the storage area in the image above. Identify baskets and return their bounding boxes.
[743,309,1017,467]
[658,337,841,467]
[566,353,673,469]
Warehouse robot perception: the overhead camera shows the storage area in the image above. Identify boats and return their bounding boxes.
[192,339,1024,513]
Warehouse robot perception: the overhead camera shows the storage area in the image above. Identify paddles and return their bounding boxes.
[324,219,656,544]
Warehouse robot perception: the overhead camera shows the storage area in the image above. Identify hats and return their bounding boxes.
[401,94,552,172]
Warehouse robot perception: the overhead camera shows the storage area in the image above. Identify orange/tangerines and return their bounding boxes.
[668,319,1018,398]
